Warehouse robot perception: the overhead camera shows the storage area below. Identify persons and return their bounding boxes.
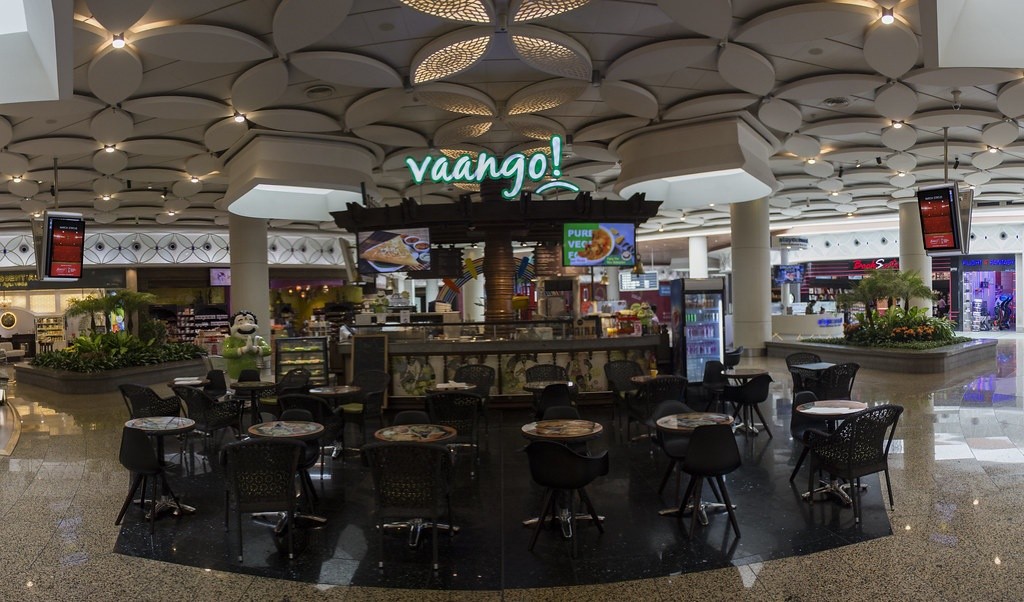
[937,294,946,318]
[806,300,825,314]
[223,311,272,390]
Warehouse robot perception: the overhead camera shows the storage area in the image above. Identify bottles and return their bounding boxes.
[644,349,656,370]
[574,312,641,339]
[67,266,71,273]
[60,231,80,246]
[686,295,715,309]
[309,319,325,336]
[272,320,288,338]
[688,326,715,338]
[687,341,717,356]
[688,358,718,382]
[212,345,217,355]
[370,316,378,325]
[600,271,609,285]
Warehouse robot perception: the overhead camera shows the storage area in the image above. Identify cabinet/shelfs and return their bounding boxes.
[275,336,331,386]
[33,316,67,355]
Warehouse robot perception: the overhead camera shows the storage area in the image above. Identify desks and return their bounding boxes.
[247,421,327,522]
[521,420,605,525]
[631,375,663,441]
[656,412,734,515]
[167,380,211,437]
[787,363,836,399]
[374,424,463,531]
[720,368,773,438]
[518,381,568,450]
[796,399,868,505]
[124,417,197,513]
[310,386,361,453]
[426,384,477,395]
[230,382,276,437]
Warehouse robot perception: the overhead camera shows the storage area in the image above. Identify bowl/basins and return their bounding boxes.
[419,253,430,262]
[574,226,615,263]
[404,236,420,245]
[413,241,430,252]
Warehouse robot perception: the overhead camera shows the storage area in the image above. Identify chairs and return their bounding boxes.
[114,351,905,569]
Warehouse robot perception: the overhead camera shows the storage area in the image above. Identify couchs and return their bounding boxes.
[0,341,25,361]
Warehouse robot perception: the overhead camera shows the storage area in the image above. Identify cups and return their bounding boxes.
[651,370,657,377]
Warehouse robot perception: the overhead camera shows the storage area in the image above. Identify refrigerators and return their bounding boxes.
[670,278,726,385]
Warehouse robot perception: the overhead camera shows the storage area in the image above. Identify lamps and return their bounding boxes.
[881,7,895,24]
[105,146,114,152]
[112,33,124,48]
[235,112,245,123]
[161,188,168,198]
[838,167,843,177]
[876,157,882,165]
[592,70,601,87]
[13,177,21,183]
[567,135,573,145]
[855,163,860,167]
[953,158,959,169]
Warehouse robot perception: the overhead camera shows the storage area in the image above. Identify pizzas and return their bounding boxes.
[359,236,420,266]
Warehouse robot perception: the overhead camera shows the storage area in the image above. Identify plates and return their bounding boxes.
[365,240,418,272]
[436,383,466,387]
